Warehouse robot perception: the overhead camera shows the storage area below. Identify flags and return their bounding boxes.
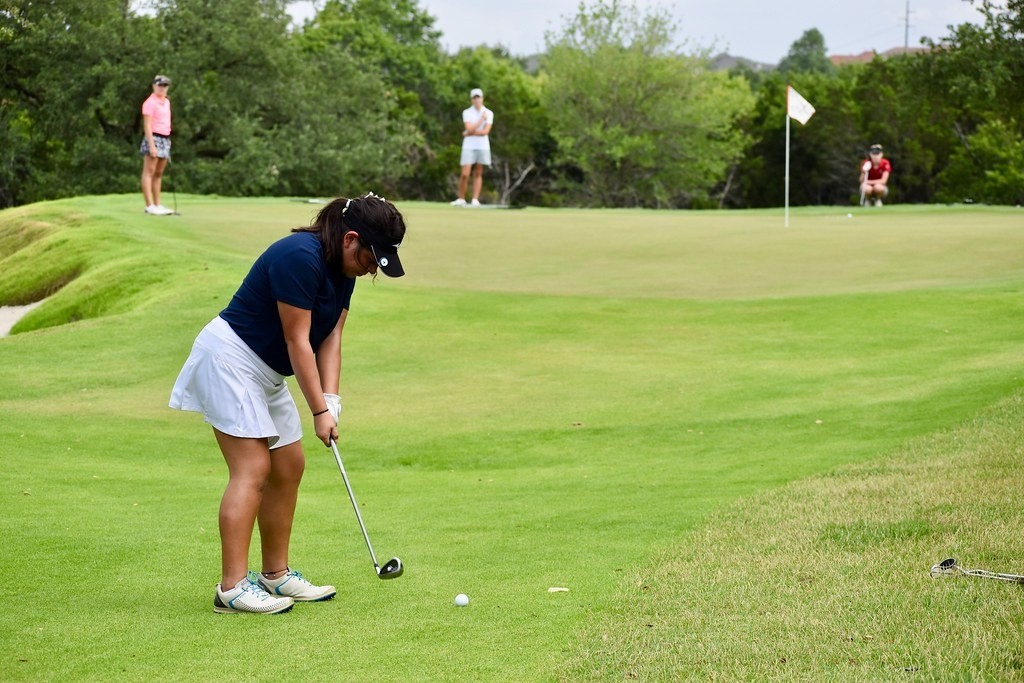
[787,85,816,126]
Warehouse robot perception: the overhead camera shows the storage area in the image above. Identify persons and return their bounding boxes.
[168,191,405,616]
[859,144,891,207]
[139,75,171,214]
[449,88,494,207]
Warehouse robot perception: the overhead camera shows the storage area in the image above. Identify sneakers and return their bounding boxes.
[258,565,335,601]
[213,577,295,614]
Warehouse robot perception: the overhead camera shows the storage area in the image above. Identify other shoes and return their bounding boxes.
[145,204,166,215]
[864,199,872,207]
[875,199,883,207]
[450,197,467,206]
[156,204,174,215]
[471,199,480,208]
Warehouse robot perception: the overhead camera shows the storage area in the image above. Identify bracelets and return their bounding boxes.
[313,408,329,417]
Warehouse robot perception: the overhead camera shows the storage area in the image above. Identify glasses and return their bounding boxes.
[158,83,168,87]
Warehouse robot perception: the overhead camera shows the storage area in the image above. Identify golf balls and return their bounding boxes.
[454,593,469,608]
[847,213,853,219]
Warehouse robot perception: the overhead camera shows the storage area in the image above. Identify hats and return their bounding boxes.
[869,146,883,154]
[341,207,405,278]
[153,75,170,84]
[470,89,483,98]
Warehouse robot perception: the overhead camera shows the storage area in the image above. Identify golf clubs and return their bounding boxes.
[859,150,872,206]
[329,435,404,580]
[168,160,182,218]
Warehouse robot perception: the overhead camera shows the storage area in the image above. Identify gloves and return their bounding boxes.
[322,393,343,424]
[863,161,871,171]
